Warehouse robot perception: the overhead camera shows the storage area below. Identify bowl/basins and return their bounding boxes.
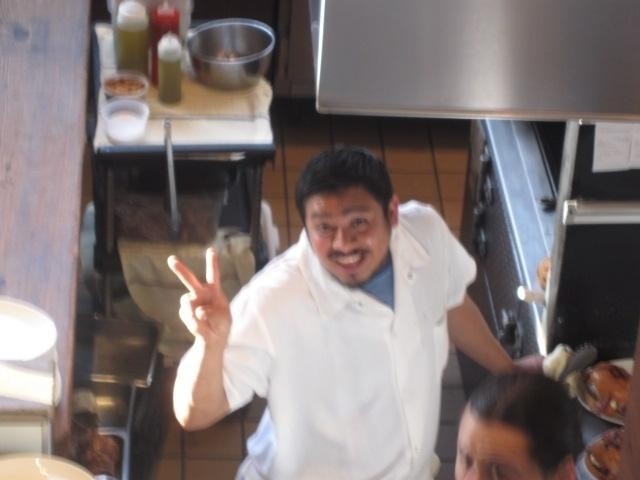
[186,17,277,91]
[100,100,151,143]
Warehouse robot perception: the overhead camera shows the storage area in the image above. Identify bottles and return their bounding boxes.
[150,2,179,89]
[156,30,183,103]
[115,2,148,75]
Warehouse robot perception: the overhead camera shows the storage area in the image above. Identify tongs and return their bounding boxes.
[555,346,598,385]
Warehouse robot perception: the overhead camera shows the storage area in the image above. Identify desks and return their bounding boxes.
[91,22,277,277]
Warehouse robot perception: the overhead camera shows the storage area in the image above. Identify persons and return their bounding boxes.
[170,143,546,480]
[454,369,585,479]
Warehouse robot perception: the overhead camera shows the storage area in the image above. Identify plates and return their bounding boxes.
[576,357,636,426]
[582,429,623,480]
[102,72,150,100]
